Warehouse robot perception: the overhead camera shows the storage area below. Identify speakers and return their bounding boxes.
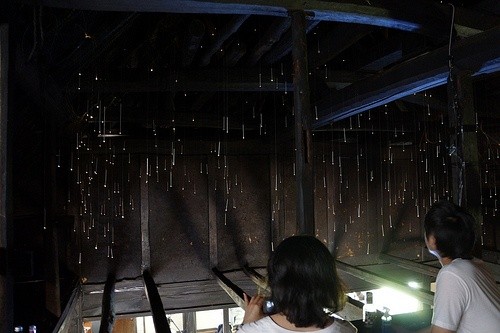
[2,208,75,333]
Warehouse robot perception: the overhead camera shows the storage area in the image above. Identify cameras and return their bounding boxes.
[262,296,276,315]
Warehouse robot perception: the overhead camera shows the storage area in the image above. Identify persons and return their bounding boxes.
[423,200,500,332]
[235,234,360,333]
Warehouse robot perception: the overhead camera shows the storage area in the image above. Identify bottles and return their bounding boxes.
[263,301,275,314]
[362,292,377,328]
[29,325,36,333]
[14,326,23,333]
